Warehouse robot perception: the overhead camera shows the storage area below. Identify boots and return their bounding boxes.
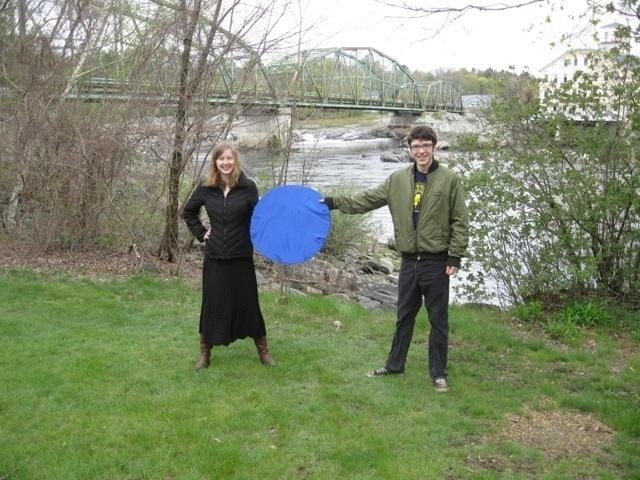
[194,333,214,372]
[254,335,277,367]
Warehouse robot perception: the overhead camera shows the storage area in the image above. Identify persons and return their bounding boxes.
[183,140,276,370]
[318,126,470,393]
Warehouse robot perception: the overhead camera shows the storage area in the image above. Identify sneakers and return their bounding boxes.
[365,367,407,379]
[433,374,451,393]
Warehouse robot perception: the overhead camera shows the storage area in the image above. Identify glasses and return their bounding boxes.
[409,143,435,153]
